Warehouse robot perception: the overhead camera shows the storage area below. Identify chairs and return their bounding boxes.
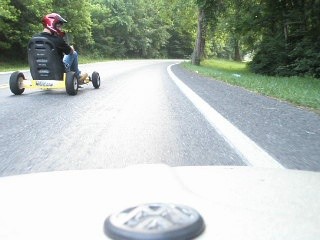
[29,37,76,80]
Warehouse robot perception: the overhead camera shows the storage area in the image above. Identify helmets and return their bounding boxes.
[41,12,68,38]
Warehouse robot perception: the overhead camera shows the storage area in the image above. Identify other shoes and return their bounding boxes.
[78,72,88,84]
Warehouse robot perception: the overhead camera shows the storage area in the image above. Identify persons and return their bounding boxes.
[40,12,89,81]
